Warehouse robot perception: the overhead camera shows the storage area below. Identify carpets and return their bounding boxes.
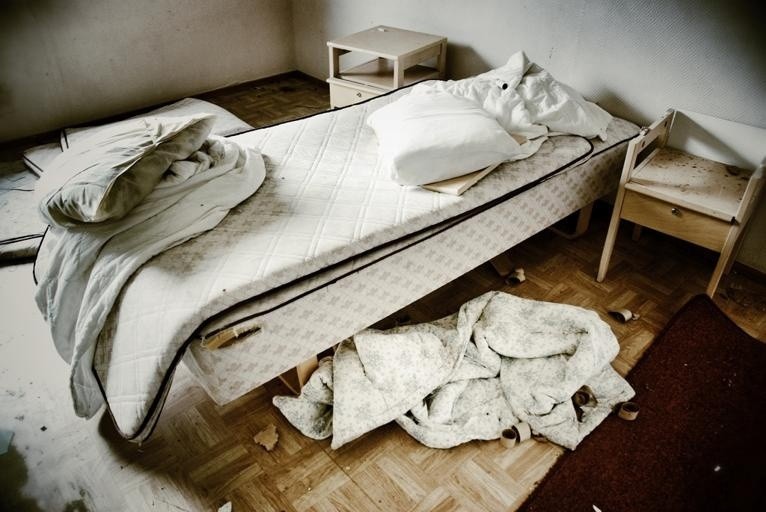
[514,293,766,511]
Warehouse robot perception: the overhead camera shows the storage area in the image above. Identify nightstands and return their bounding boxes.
[326,24,447,110]
[597,107,766,299]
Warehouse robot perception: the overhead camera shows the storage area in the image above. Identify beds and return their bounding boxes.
[33,78,642,443]
